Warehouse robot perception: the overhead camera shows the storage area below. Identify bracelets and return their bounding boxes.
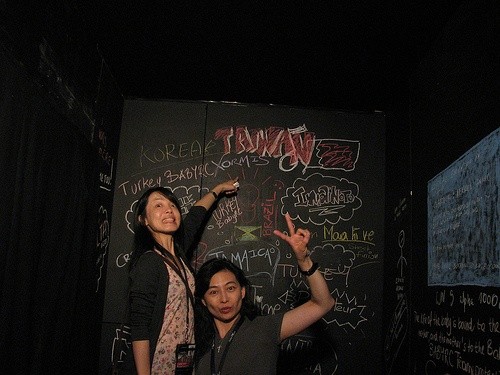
[298,259,320,277]
[208,190,219,201]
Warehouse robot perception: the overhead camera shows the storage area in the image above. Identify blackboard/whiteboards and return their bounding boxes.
[88,101,122,303]
[99,97,385,374]
[389,102,500,375]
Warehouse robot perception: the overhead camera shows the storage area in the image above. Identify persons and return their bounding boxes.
[190,213,337,375]
[127,176,241,375]
